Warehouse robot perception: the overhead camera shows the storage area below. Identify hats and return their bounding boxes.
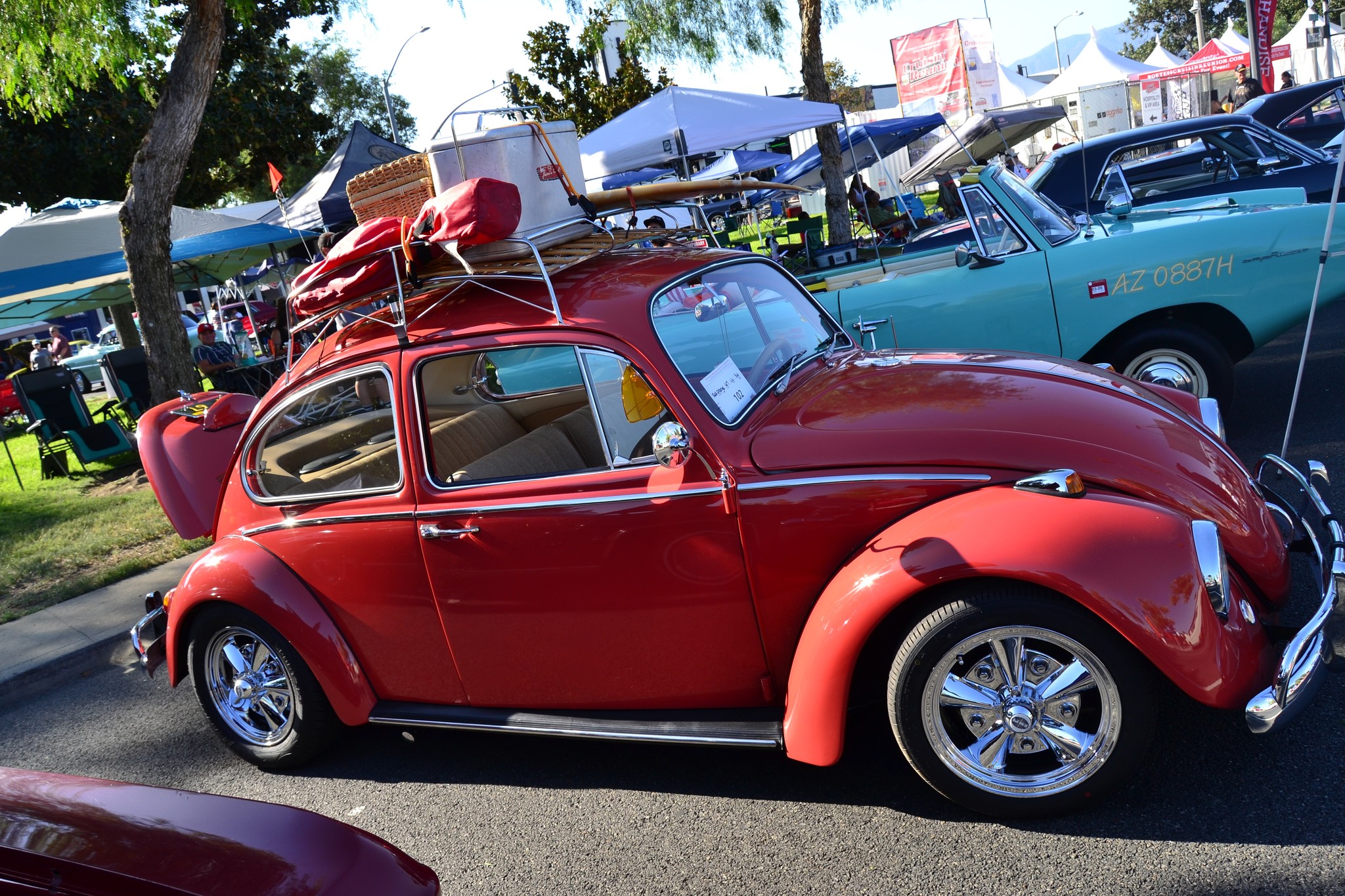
[1235,64,1246,72]
[197,323,216,334]
[1004,151,1019,162]
[32,339,40,345]
[1052,143,1066,151]
[644,216,665,229]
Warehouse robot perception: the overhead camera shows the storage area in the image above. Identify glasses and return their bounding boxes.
[34,344,39,346]
[648,226,663,229]
[49,330,56,333]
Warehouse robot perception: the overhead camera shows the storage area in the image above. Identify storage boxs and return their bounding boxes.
[786,204,803,219]
[344,152,436,226]
[811,239,858,269]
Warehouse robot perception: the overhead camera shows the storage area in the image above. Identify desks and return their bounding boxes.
[729,209,758,239]
[223,352,302,400]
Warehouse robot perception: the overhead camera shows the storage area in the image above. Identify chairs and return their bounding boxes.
[756,198,785,230]
[99,344,156,435]
[857,208,908,248]
[893,192,940,231]
[847,192,899,240]
[192,343,240,392]
[934,172,983,221]
[12,363,140,482]
[768,214,827,276]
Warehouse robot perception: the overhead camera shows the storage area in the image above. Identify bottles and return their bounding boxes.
[231,345,242,368]
[303,333,310,349]
[268,338,276,356]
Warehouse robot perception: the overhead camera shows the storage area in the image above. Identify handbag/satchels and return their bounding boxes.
[930,211,949,223]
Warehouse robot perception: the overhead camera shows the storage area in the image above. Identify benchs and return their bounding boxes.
[280,403,528,497]
[446,424,590,482]
[545,390,675,468]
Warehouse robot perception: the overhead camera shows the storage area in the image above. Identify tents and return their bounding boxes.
[0,7,1345,495]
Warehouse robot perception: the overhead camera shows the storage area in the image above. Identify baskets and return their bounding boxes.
[345,152,435,227]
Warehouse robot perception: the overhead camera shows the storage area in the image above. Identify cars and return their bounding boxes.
[5,338,94,379]
[484,163,1345,423]
[1100,73,1345,191]
[56,310,225,394]
[196,299,279,340]
[896,112,1345,253]
[128,117,1345,820]
[693,193,744,232]
